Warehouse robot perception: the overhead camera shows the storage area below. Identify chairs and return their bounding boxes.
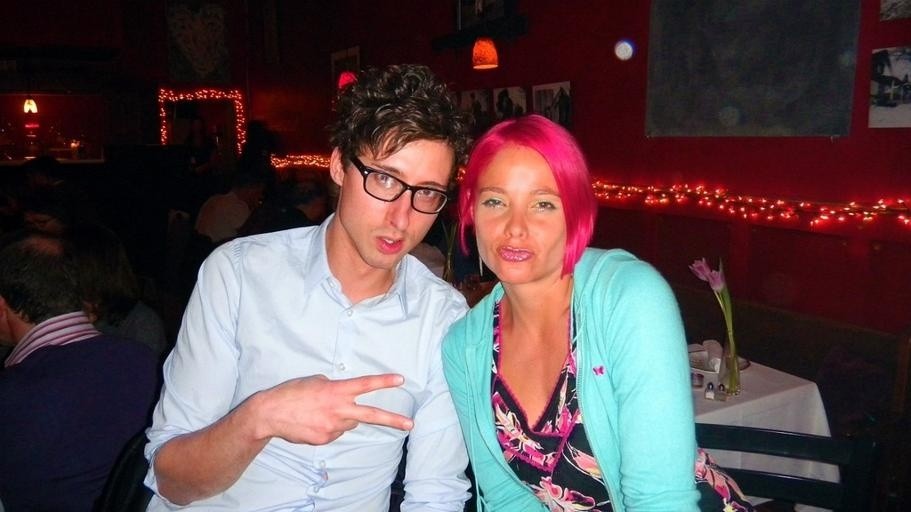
[695,422,876,511]
[94,422,153,510]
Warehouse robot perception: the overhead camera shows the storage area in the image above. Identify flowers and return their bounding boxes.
[688,255,749,391]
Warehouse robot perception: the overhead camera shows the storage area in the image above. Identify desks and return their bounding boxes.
[684,342,843,512]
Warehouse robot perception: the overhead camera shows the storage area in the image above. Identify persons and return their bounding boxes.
[2,117,336,510]
[140,60,476,510]
[440,114,757,510]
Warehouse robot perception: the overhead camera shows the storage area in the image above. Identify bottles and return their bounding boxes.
[689,372,728,402]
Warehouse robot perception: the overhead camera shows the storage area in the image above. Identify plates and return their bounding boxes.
[689,355,750,374]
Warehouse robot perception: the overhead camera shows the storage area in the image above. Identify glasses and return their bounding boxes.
[346,148,449,214]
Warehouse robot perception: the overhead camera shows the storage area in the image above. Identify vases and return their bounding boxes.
[717,329,743,398]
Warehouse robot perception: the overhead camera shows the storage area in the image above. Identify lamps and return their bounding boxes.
[470,0,499,70]
